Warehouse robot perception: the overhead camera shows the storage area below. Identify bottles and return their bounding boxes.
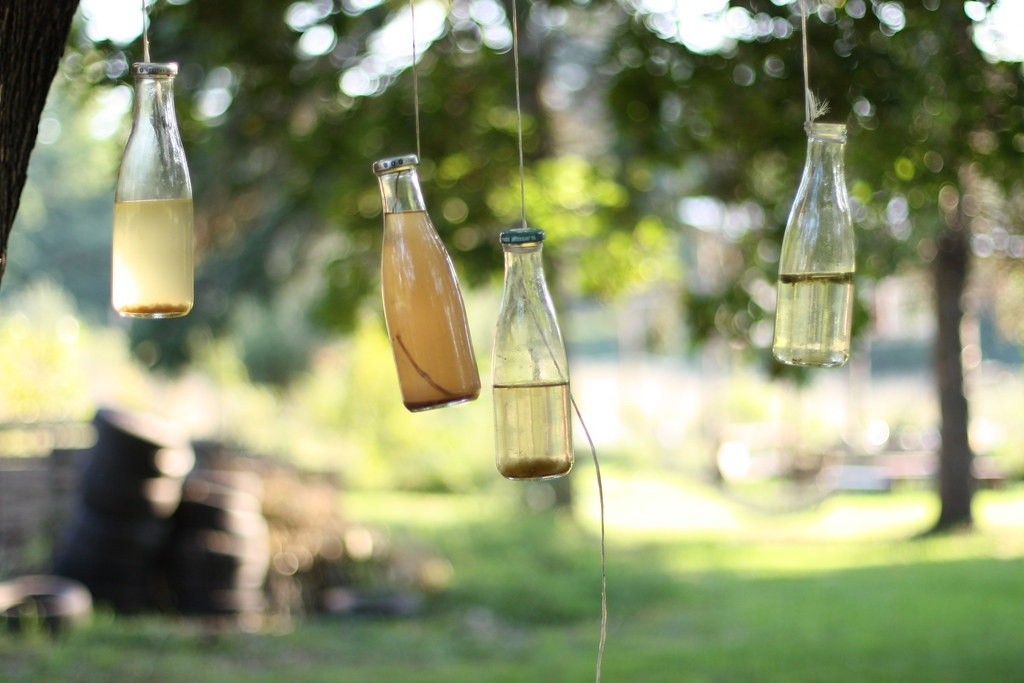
[493,227,572,479]
[374,152,481,413]
[112,61,193,318]
[772,122,855,367]
